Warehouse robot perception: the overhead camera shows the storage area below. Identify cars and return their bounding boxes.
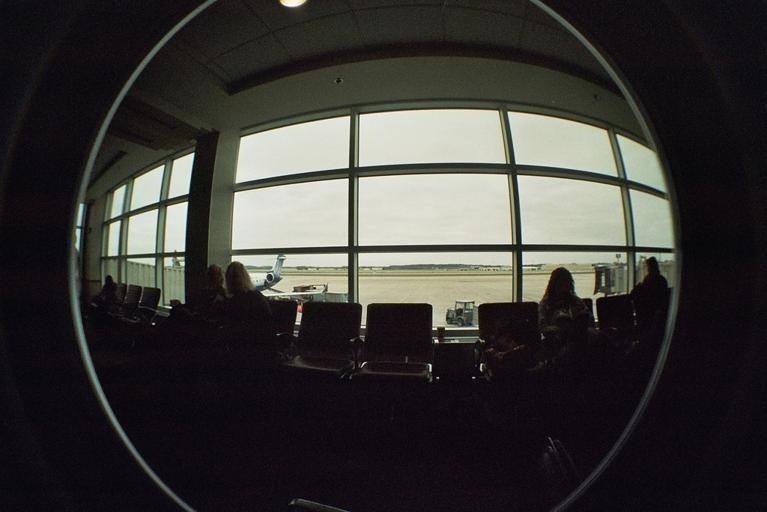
[446,300,474,327]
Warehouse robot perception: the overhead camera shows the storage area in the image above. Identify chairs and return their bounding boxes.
[265,297,435,384]
[92,282,162,348]
[477,288,674,388]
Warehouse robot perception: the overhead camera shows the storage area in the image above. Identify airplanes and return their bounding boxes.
[250,255,287,293]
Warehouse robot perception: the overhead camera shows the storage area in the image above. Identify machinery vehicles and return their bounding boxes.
[292,283,328,312]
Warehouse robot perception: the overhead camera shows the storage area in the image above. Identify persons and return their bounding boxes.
[537,267,594,342]
[99,274,118,298]
[170,261,274,326]
[635,256,668,292]
